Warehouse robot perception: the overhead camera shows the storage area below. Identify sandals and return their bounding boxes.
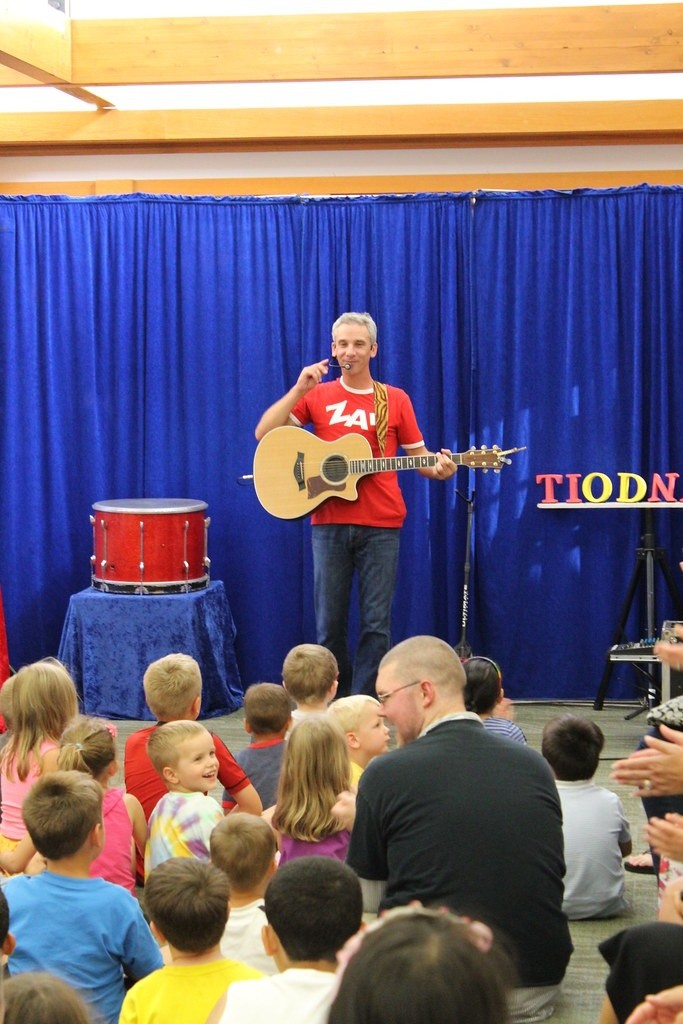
[624,849,656,874]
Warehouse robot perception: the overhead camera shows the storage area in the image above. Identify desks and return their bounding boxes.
[57,579,244,721]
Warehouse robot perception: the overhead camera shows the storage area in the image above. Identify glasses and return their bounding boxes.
[378,680,422,703]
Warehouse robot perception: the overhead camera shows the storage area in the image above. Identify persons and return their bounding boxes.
[599,563,683,1024]
[123,642,391,886]
[0,886,16,1024]
[462,655,528,745]
[252,312,456,705]
[2,770,164,1024]
[349,637,575,1023]
[0,972,96,1024]
[320,901,512,1024]
[118,813,366,1024]
[0,656,148,898]
[541,713,632,920]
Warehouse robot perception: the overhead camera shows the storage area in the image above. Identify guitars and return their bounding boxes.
[252,424,528,522]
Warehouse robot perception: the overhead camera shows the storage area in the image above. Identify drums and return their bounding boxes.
[88,497,211,595]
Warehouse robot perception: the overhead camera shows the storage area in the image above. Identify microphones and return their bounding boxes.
[328,358,351,370]
